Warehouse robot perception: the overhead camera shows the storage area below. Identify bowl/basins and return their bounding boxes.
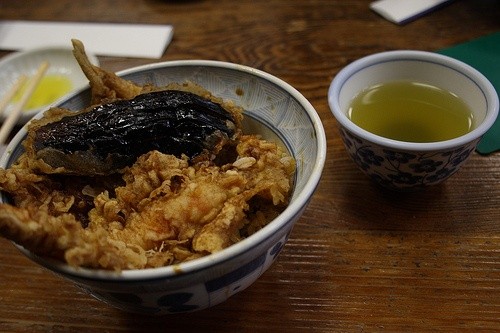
[328,51,499,192]
[0,58,327,318]
[0,44,100,124]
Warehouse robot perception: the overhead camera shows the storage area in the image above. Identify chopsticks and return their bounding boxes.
[1,58,53,154]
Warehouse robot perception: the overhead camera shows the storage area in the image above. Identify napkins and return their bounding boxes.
[369,0,447,26]
[0,19,174,59]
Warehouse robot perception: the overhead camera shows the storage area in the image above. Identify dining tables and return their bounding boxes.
[0,0,500,333]
